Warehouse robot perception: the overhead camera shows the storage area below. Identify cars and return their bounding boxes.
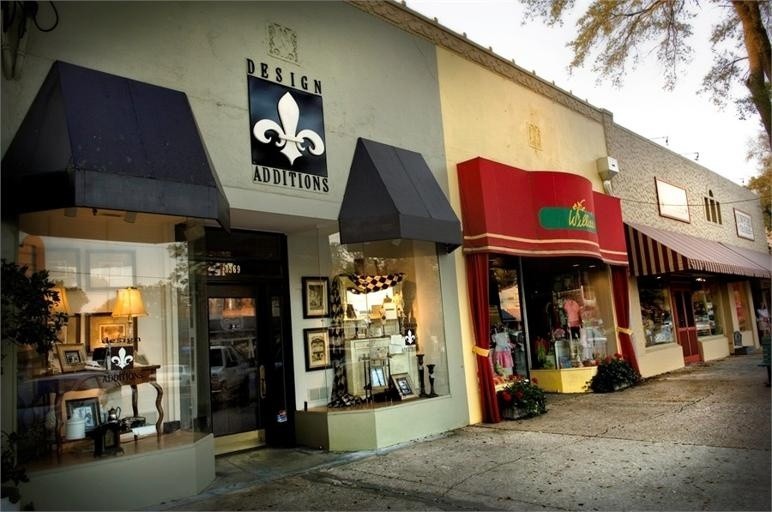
[157,364,195,392]
[210,345,249,410]
[586,326,608,345]
[18,373,124,427]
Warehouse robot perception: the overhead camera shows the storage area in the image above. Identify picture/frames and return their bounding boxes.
[51,313,137,374]
[64,395,101,433]
[41,246,138,291]
[389,372,419,401]
[301,274,332,372]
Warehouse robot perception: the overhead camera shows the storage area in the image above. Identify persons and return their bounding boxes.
[757,302,770,337]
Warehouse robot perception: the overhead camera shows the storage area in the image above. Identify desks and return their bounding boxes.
[31,365,164,463]
[342,336,421,401]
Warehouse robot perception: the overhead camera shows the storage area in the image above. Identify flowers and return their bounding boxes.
[591,354,640,393]
[494,374,545,417]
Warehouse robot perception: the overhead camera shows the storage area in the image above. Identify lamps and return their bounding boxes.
[41,285,74,376]
[110,287,145,348]
